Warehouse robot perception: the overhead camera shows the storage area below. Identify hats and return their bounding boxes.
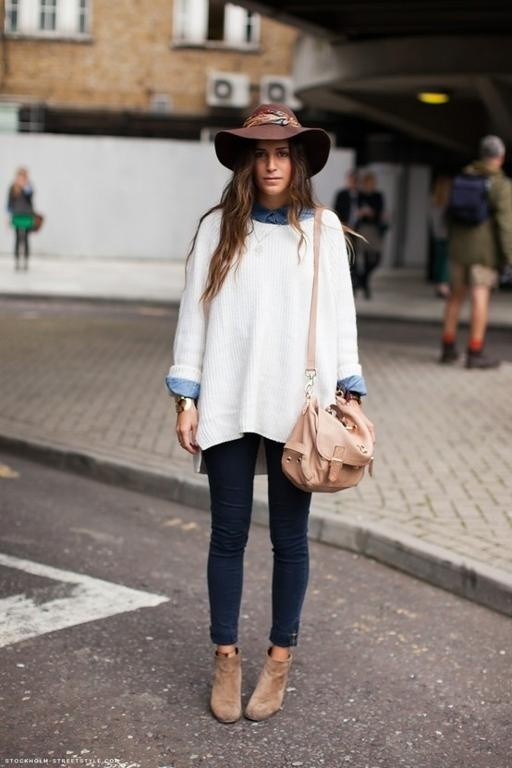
[480,135,504,157]
[214,102,331,179]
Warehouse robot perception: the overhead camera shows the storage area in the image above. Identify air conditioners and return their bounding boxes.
[205,70,250,109]
[260,74,294,108]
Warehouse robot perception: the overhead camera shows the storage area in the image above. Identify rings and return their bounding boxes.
[179,441,181,444]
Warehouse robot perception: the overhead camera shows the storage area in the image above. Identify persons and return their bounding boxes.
[6,168,33,270]
[438,133,511,370]
[356,174,391,300]
[426,175,512,297]
[162,100,366,724]
[334,173,373,296]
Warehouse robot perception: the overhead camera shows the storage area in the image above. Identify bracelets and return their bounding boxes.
[174,396,194,413]
[349,394,361,405]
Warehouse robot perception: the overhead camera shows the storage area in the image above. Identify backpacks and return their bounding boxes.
[445,174,492,225]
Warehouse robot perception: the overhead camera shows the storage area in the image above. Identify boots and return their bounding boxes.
[206,642,243,724]
[245,646,292,721]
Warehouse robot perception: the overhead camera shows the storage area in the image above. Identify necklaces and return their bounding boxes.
[247,210,292,254]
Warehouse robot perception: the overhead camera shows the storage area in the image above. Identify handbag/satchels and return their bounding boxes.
[32,213,43,232]
[281,392,375,493]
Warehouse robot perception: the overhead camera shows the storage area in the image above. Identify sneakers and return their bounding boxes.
[437,341,460,365]
[463,349,501,369]
